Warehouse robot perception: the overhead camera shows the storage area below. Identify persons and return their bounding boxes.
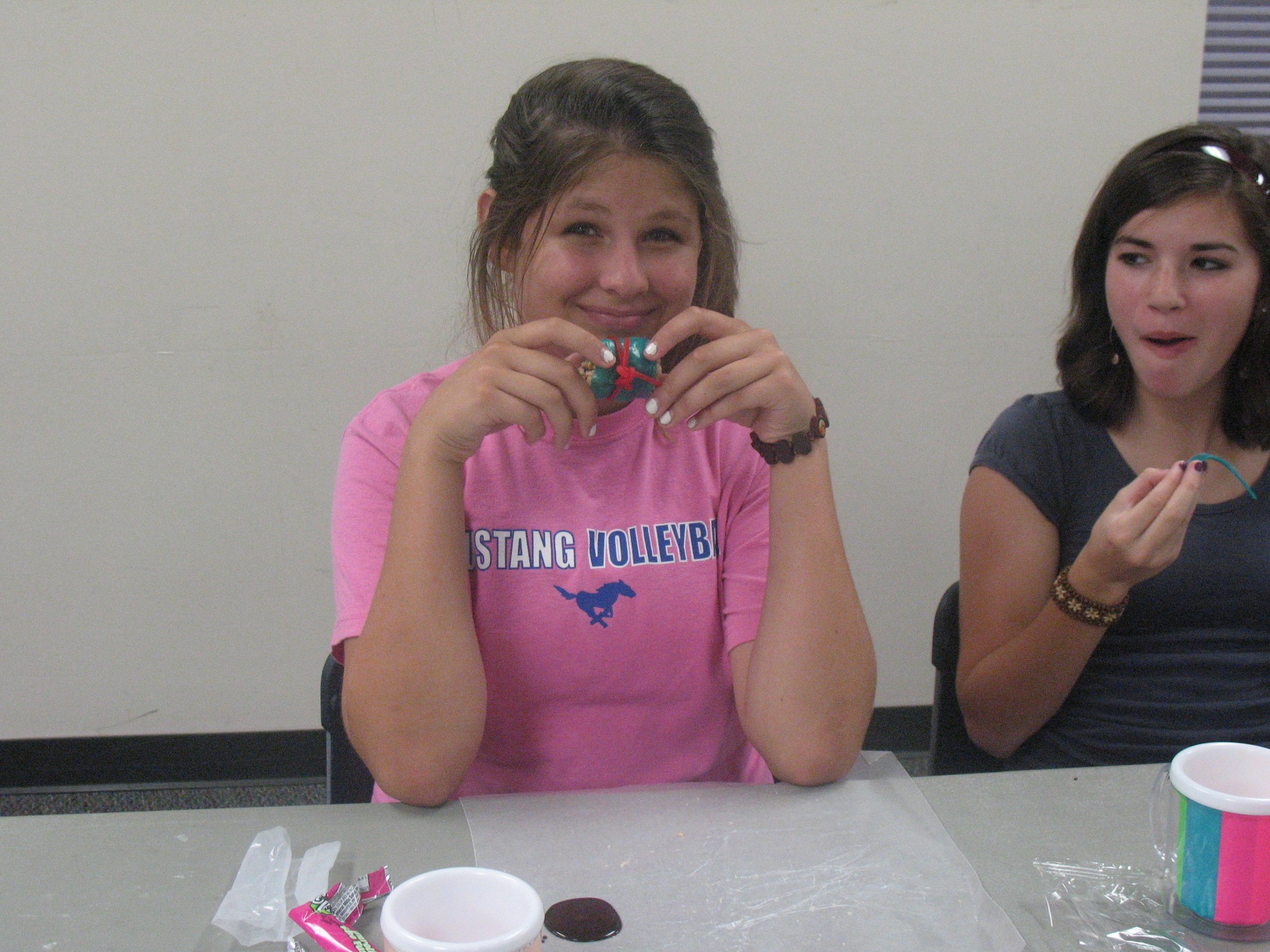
[325,58,879,812]
[953,122,1270,770]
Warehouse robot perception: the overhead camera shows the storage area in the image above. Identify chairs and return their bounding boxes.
[321,653,375,804]
[928,581,1011,776]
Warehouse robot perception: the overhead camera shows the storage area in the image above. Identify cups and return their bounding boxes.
[381,867,545,952]
[1147,742,1270,942]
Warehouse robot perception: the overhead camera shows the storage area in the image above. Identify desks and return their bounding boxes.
[0,762,1270,952]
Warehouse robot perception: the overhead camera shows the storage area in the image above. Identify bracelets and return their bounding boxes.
[1050,567,1130,624]
[753,393,832,465]
